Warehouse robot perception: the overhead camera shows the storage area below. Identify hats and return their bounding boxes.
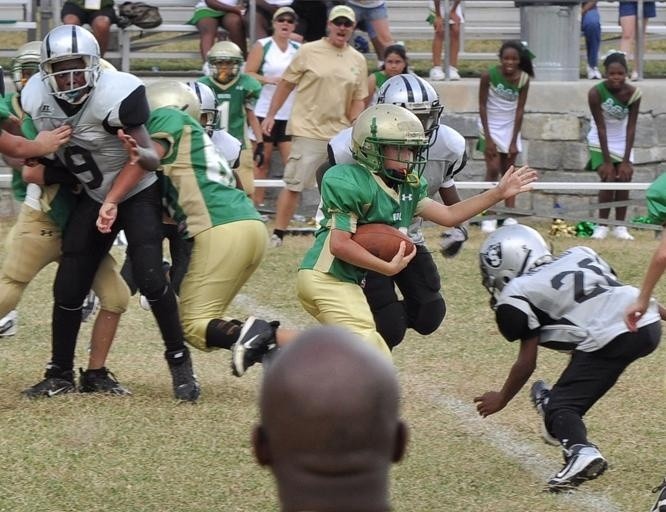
[273,7,295,21]
[329,5,355,22]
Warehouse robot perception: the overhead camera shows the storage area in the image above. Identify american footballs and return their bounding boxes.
[352,224,416,262]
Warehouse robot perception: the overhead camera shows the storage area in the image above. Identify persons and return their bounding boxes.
[187,1,417,199]
[584,52,643,241]
[326,73,468,351]
[625,174,665,511]
[259,5,371,246]
[617,1,657,81]
[580,2,602,80]
[426,1,466,81]
[59,1,122,58]
[473,224,664,491]
[475,40,535,230]
[229,104,537,364]
[249,327,408,511]
[0,23,270,401]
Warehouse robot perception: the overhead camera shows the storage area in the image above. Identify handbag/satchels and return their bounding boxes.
[118,3,161,28]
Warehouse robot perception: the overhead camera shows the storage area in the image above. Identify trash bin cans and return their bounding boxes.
[514,0,581,82]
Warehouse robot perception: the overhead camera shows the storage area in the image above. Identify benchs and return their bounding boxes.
[360,0,521,81]
[52,0,256,73]
[583,1,665,80]
[0,0,37,58]
[279,175,653,230]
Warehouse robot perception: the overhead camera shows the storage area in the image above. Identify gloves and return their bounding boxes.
[439,225,469,255]
[253,143,264,167]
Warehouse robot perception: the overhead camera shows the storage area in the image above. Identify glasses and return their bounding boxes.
[331,18,352,27]
[278,17,294,23]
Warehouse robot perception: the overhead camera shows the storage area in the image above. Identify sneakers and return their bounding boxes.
[78,365,133,396]
[590,226,610,240]
[429,65,445,80]
[19,362,75,399]
[80,288,100,322]
[502,217,517,225]
[480,219,497,232]
[449,65,461,80]
[165,345,200,402]
[530,380,666,512]
[231,316,278,378]
[613,226,634,240]
[139,258,170,311]
[0,310,17,336]
[586,63,602,79]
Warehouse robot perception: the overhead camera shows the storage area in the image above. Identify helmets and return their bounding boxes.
[12,23,244,138]
[349,103,429,184]
[376,74,444,152]
[480,224,555,292]
